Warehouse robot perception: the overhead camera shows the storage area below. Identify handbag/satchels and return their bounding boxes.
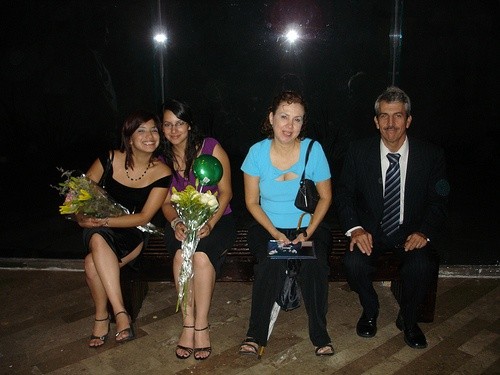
[276,259,302,312]
[294,179,320,214]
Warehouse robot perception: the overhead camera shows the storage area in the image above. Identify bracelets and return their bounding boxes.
[171,218,183,231]
[105,220,108,228]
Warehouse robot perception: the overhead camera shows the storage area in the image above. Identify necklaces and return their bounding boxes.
[127,162,150,181]
[174,154,184,171]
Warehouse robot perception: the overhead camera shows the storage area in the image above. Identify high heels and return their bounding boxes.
[176,325,194,359]
[113,311,134,343]
[194,322,212,359]
[89,311,110,347]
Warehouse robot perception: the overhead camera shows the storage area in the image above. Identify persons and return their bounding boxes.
[76,111,173,349]
[238,89,336,355]
[155,99,233,361]
[335,86,449,348]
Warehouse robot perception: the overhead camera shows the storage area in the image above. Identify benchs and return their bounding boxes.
[106,220,438,324]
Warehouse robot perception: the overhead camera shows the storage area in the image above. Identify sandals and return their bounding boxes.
[314,344,335,355]
[239,341,259,357]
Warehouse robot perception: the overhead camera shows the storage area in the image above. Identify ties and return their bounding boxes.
[382,153,401,237]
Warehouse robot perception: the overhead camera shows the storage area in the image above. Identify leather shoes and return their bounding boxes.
[395,309,427,348]
[356,316,377,338]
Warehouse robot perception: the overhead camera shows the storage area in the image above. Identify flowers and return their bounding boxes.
[168,183,219,313]
[51,166,161,235]
